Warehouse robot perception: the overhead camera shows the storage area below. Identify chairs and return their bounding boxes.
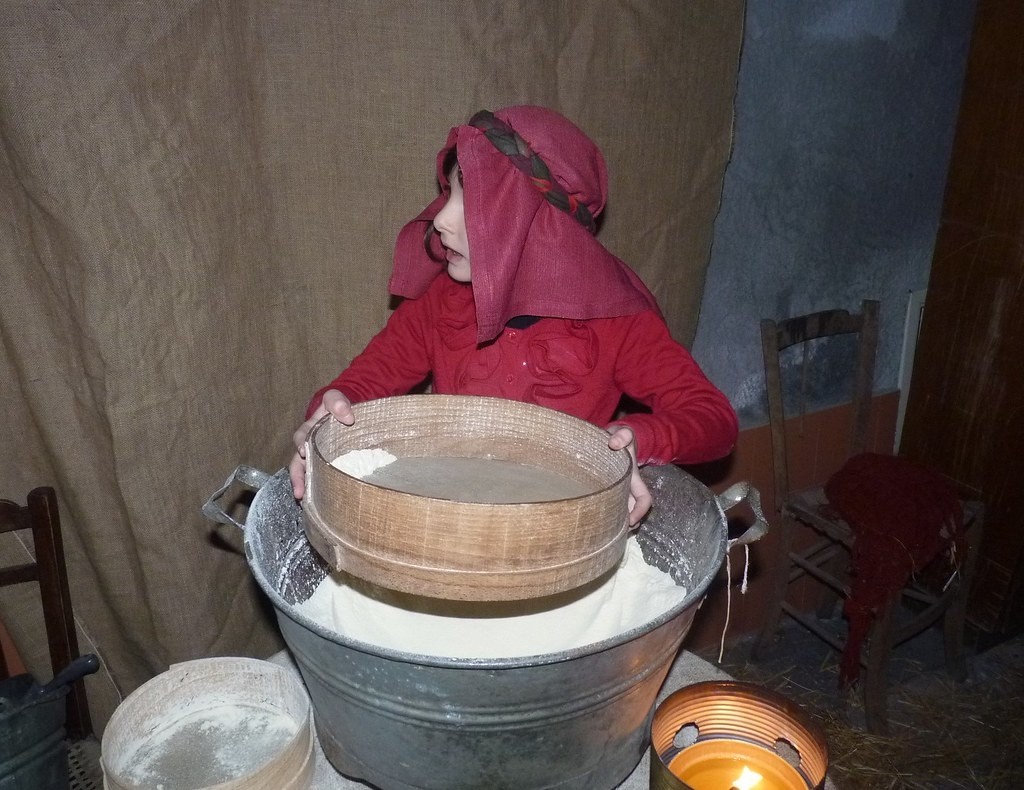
[760,297,990,744]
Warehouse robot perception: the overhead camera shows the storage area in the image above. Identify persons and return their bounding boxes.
[287,104,739,533]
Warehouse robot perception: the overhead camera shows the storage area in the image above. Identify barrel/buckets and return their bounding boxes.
[200,460,769,790]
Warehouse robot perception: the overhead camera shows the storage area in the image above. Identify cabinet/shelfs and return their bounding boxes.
[900,0,1024,656]
